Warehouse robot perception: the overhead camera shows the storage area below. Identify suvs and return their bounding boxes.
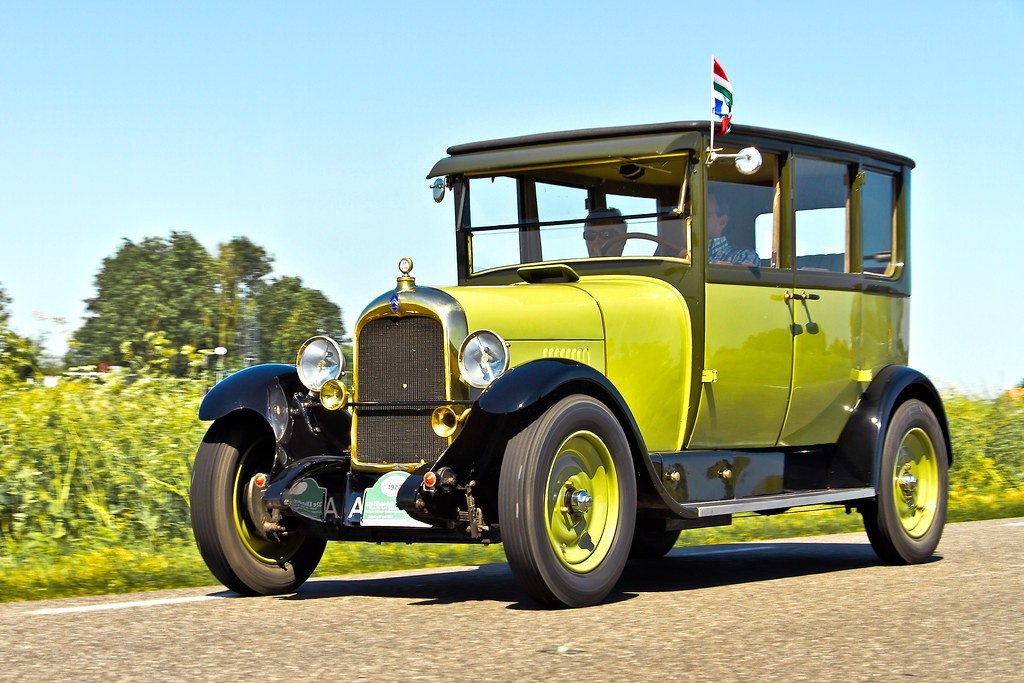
[189,119,955,611]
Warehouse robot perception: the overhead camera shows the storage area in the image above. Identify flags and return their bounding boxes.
[714,58,733,136]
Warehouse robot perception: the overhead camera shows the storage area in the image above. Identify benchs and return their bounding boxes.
[760,254,845,271]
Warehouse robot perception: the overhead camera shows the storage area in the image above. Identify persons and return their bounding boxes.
[662,191,760,266]
[584,208,627,258]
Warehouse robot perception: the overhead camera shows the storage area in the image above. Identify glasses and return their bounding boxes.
[583,230,618,240]
